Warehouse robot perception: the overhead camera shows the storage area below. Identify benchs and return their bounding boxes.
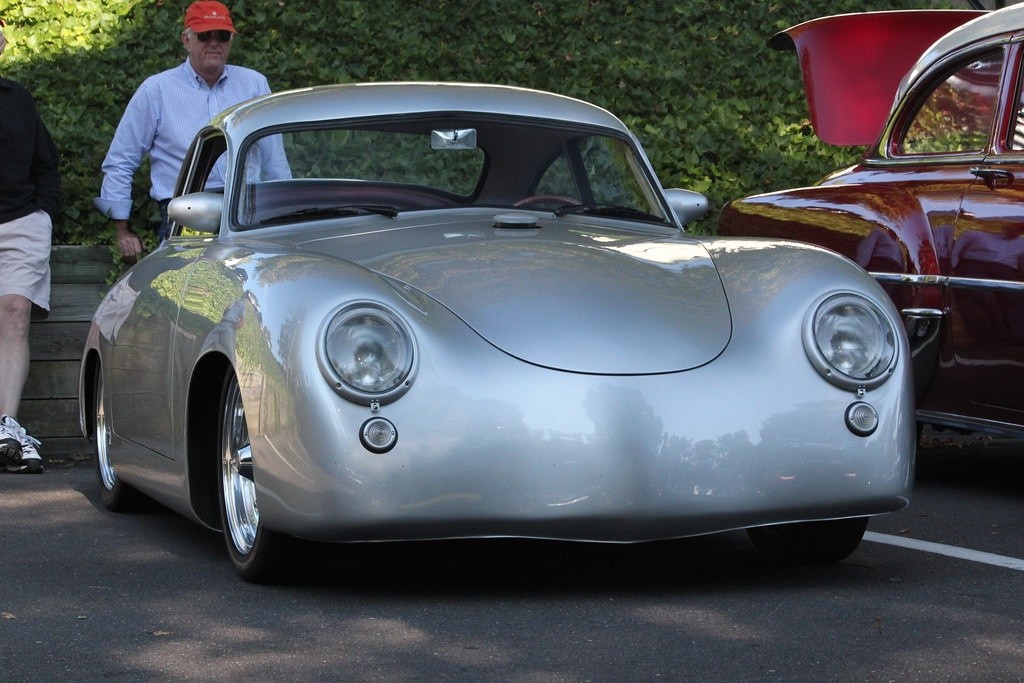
[211,178,458,227]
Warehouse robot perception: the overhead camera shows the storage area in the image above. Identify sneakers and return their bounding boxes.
[0,414,46,473]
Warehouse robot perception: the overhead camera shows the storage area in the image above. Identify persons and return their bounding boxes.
[93,0,293,257]
[0,74,61,472]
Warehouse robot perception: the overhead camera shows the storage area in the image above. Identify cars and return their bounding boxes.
[717,3,1024,443]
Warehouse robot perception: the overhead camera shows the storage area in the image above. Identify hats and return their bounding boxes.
[183,0,238,36]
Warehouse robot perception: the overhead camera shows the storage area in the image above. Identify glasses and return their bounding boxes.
[189,30,233,43]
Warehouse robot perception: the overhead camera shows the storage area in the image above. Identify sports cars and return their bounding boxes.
[77,80,914,587]
